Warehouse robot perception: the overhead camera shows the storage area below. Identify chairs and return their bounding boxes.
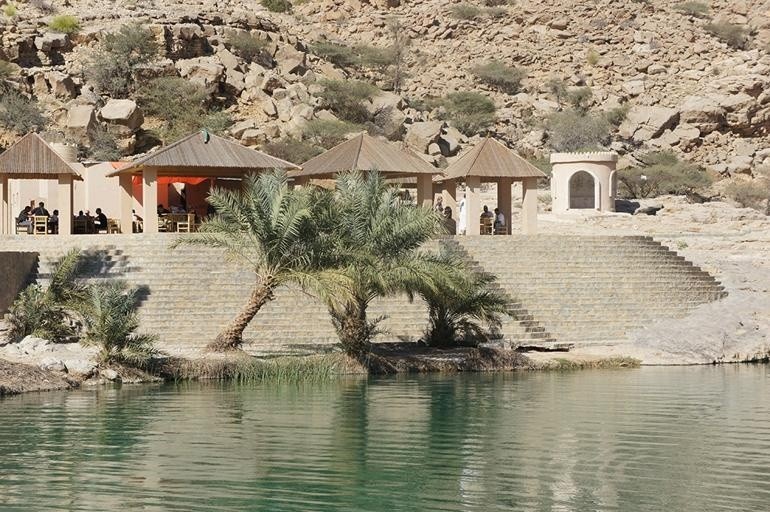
[480,215,509,236]
[14,216,121,236]
[131,210,205,233]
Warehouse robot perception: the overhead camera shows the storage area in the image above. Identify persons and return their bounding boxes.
[48,209,60,234]
[132,209,143,233]
[459,193,466,235]
[403,190,411,203]
[434,195,444,234]
[94,207,107,233]
[157,204,200,231]
[481,205,493,234]
[493,208,506,235]
[76,210,87,228]
[16,206,34,234]
[29,201,51,231]
